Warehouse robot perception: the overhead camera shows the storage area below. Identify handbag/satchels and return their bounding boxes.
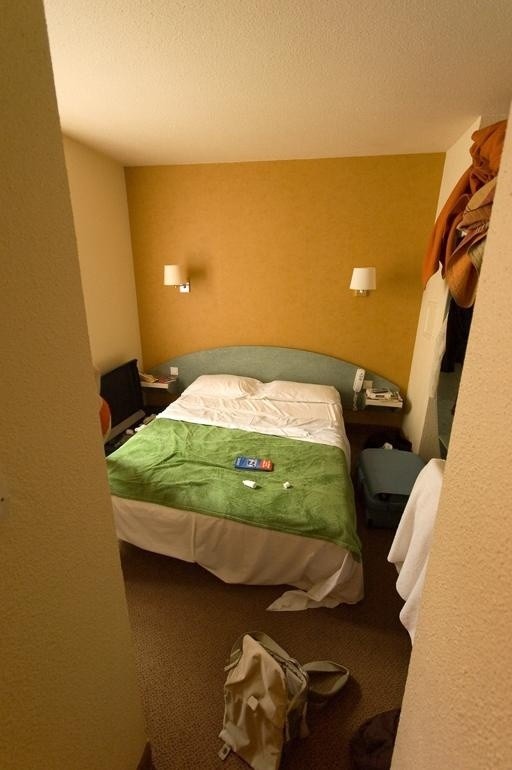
[217,631,350,770]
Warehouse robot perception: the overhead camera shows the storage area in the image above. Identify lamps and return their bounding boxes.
[162,263,194,295]
[350,267,377,301]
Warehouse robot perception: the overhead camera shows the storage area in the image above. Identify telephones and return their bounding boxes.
[352,368,366,393]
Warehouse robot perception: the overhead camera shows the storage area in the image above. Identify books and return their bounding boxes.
[234,455,274,471]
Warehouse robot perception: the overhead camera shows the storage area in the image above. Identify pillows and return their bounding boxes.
[184,375,261,400]
[260,377,338,405]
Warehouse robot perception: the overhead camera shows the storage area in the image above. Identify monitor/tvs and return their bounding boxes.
[100,359,146,445]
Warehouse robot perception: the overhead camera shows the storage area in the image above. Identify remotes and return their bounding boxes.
[142,415,155,425]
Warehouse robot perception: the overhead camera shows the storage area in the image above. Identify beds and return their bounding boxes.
[106,344,402,613]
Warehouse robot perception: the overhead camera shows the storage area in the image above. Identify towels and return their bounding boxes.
[384,458,447,647]
